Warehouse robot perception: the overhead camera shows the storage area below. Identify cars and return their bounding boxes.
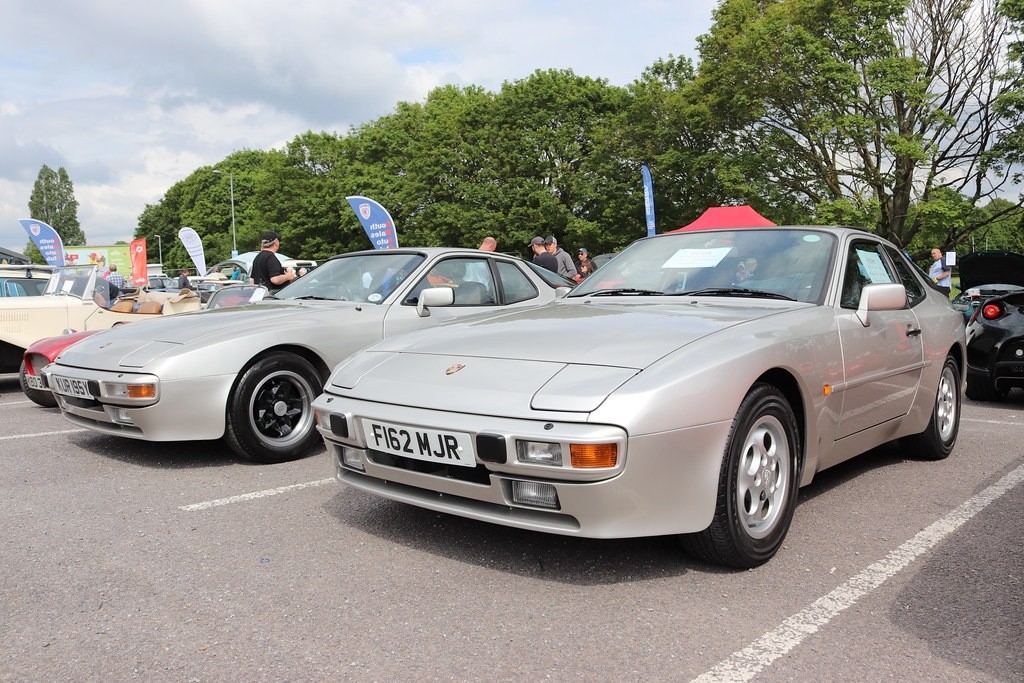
[950,249,1024,403]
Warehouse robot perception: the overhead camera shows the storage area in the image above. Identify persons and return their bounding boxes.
[206,265,228,280]
[229,265,241,280]
[927,248,952,298]
[479,236,497,252]
[178,266,192,291]
[249,231,294,295]
[574,248,598,279]
[104,264,125,305]
[544,236,577,279]
[527,236,558,273]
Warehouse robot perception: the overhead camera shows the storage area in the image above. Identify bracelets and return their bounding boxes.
[937,277,939,280]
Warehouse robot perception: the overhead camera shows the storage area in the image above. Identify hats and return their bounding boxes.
[545,236,557,244]
[261,232,280,244]
[578,248,587,253]
[527,237,545,247]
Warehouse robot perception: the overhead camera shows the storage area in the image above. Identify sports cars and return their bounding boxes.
[0,247,319,409]
[39,246,581,467]
[309,224,968,570]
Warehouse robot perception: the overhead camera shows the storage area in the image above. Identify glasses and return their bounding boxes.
[578,253,583,255]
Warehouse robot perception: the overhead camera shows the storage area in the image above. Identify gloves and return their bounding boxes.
[933,278,938,284]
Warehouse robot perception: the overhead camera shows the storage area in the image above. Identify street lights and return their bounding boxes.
[211,170,236,251]
[154,234,162,264]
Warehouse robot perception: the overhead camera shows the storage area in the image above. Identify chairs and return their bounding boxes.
[811,248,859,306]
[112,301,132,312]
[136,301,162,314]
[456,282,488,304]
